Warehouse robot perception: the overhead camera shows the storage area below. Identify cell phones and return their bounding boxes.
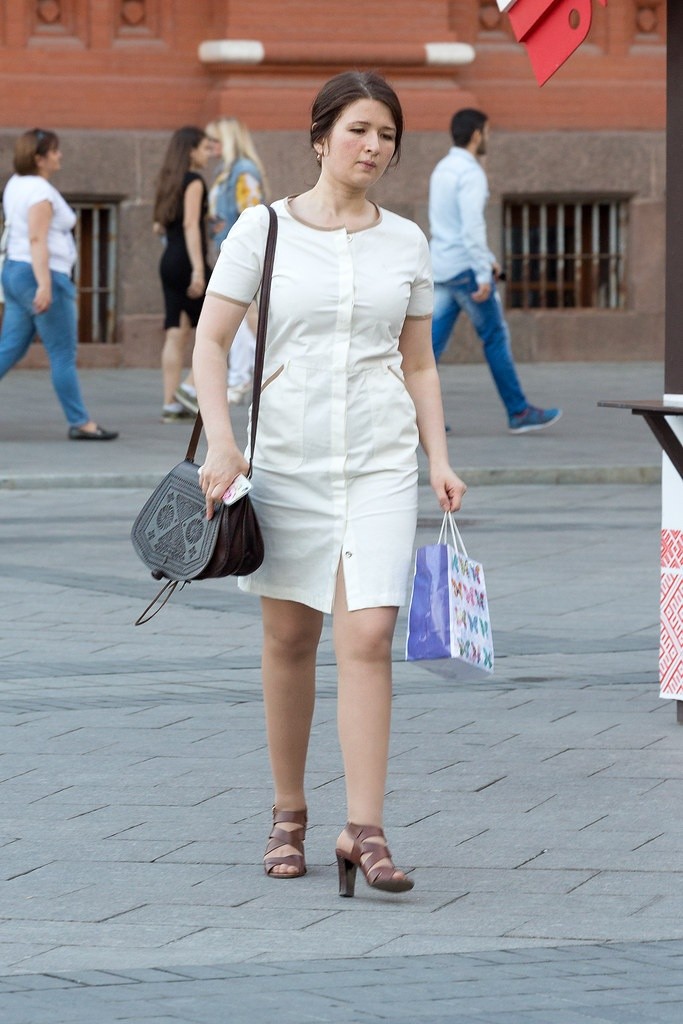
[222,473,253,507]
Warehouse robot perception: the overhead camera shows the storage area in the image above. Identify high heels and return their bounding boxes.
[335,823,414,897]
[264,804,307,878]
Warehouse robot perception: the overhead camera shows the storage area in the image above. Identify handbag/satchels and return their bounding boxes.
[0,253,5,304]
[405,511,494,682]
[130,460,264,626]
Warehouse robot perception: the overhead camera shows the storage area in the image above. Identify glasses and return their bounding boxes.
[36,130,44,141]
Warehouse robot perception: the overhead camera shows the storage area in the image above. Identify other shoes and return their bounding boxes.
[226,387,244,405]
[69,424,119,440]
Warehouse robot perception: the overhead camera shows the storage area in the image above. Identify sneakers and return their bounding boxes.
[507,405,563,434]
[173,385,199,418]
[161,408,197,425]
[445,425,452,435]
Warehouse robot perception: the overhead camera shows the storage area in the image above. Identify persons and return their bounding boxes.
[200,119,274,404]
[429,108,561,437]
[0,129,120,442]
[192,71,468,898]
[153,125,214,423]
